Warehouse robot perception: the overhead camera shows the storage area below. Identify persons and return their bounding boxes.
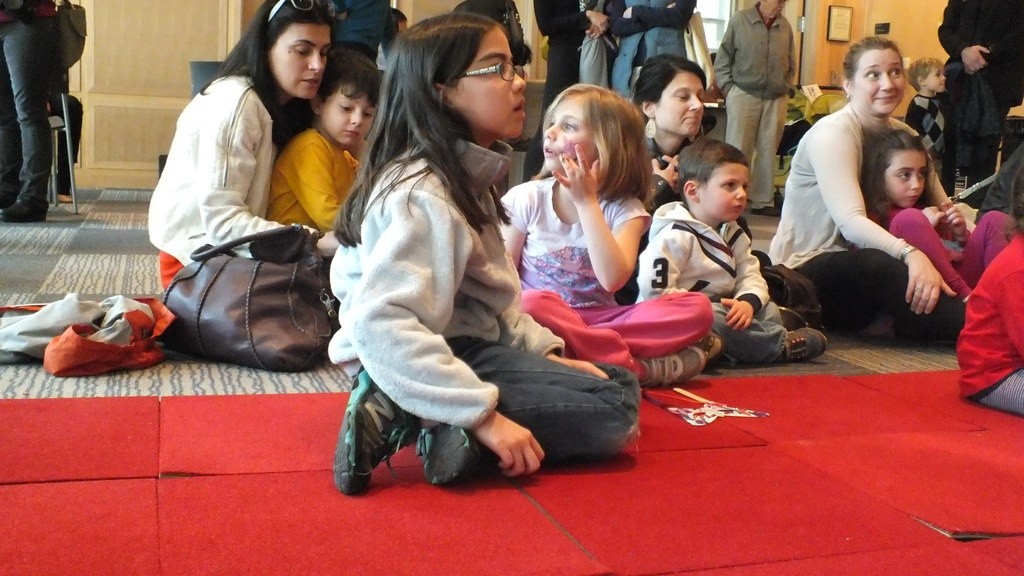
[635,138,829,366]
[523,0,697,183]
[501,82,714,385]
[957,165,1024,413]
[615,51,752,306]
[904,57,946,175]
[328,12,641,492]
[939,0,1024,209]
[770,36,1013,348]
[0,0,57,221]
[148,0,407,290]
[714,0,798,216]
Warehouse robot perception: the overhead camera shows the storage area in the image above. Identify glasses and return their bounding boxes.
[451,62,526,82]
[267,1,336,26]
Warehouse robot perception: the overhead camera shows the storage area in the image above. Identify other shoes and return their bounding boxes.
[752,207,780,217]
[782,327,828,364]
[698,335,723,360]
[56,194,72,203]
[1,199,47,222]
[640,346,706,388]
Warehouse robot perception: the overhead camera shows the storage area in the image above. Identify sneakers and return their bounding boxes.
[416,422,482,484]
[333,365,422,495]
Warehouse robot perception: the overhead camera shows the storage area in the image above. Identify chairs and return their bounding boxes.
[49,93,79,215]
[770,94,847,208]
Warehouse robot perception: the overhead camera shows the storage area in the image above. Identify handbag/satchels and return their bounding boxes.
[516,39,533,66]
[52,2,87,82]
[684,11,715,90]
[540,36,550,60]
[751,250,824,331]
[161,226,340,374]
